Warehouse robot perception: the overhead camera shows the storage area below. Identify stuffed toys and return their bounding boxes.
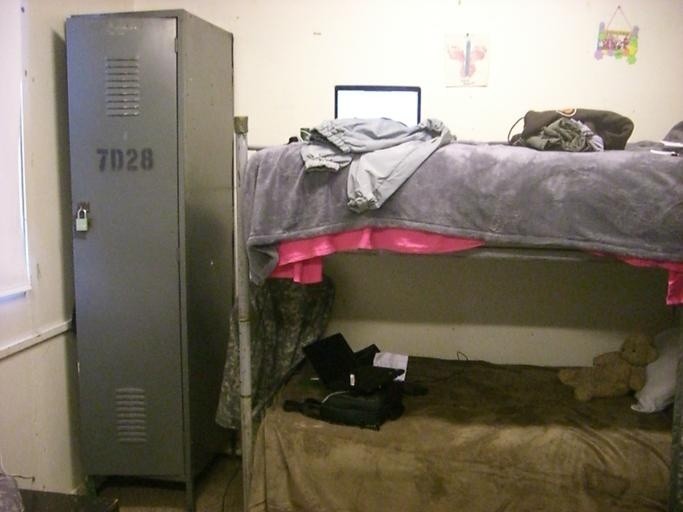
[557,332,660,401]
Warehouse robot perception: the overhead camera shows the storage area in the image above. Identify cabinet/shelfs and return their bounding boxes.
[64,10,235,512]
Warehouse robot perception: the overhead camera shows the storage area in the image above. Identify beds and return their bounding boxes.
[235,116,682,510]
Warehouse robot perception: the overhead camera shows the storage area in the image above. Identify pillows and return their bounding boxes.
[631,326,683,413]
